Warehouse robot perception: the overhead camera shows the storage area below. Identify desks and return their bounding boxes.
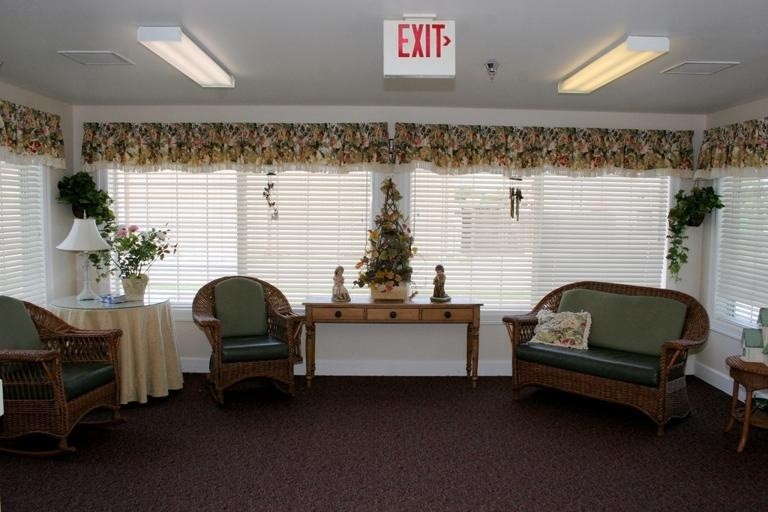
[46,295,185,405]
[301,295,484,389]
[723,355,768,452]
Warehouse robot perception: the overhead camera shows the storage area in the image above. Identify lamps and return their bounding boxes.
[135,25,235,89]
[56,210,112,301]
[557,35,670,95]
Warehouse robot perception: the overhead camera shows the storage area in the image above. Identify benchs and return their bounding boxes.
[502,281,710,437]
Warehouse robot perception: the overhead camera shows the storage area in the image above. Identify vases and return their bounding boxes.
[370,282,415,303]
[122,275,149,301]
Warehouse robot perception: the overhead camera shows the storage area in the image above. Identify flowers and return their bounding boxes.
[77,219,178,283]
[352,176,419,301]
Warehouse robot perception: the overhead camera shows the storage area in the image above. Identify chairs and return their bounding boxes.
[0,295,130,473]
[191,275,306,405]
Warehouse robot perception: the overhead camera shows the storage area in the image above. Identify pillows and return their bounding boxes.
[528,309,593,351]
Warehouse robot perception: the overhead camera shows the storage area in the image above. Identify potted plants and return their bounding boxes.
[665,186,725,284]
[56,172,116,226]
